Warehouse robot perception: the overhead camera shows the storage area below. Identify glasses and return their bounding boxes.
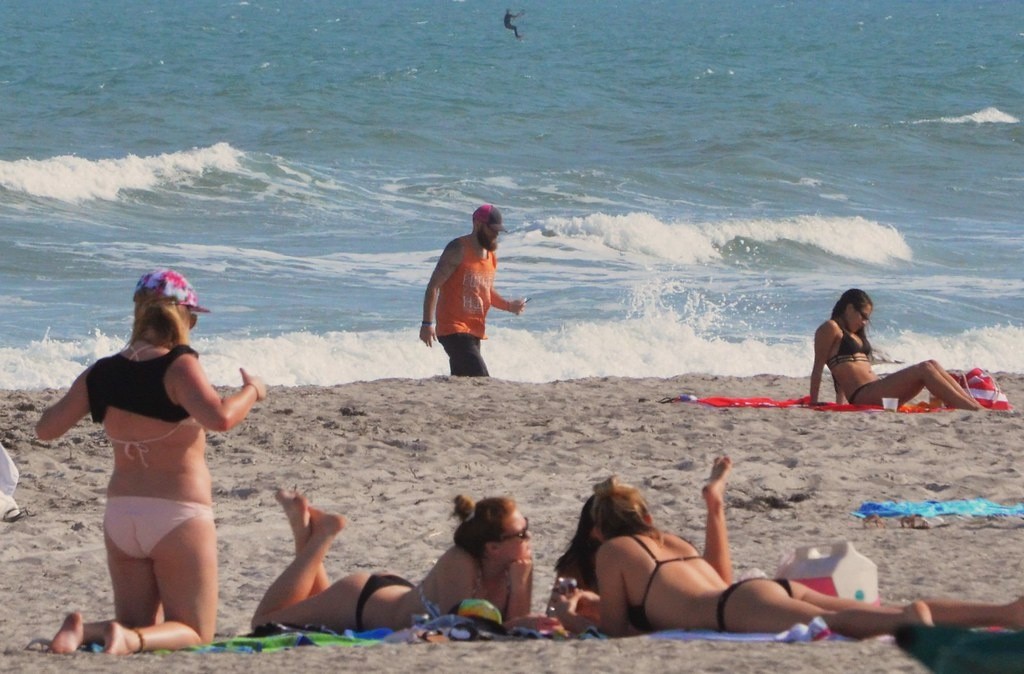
[184,305,197,329]
[852,304,871,323]
[502,516,530,541]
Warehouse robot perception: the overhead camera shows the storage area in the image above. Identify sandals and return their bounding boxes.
[4,507,38,521]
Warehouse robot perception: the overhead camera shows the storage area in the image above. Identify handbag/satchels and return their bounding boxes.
[946,368,1014,411]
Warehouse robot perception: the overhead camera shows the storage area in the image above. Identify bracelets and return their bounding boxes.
[508,302,509,311]
[245,383,259,400]
[422,321,432,327]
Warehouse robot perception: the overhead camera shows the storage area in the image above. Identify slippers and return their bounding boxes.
[447,622,481,641]
[638,395,675,404]
[418,628,449,642]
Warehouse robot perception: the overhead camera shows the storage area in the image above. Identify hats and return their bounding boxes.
[473,205,505,232]
[133,268,210,312]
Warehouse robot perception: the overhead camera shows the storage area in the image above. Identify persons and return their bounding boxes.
[546,456,731,613]
[420,205,526,375]
[36,267,265,655]
[809,289,984,409]
[250,489,565,634]
[561,478,1024,638]
